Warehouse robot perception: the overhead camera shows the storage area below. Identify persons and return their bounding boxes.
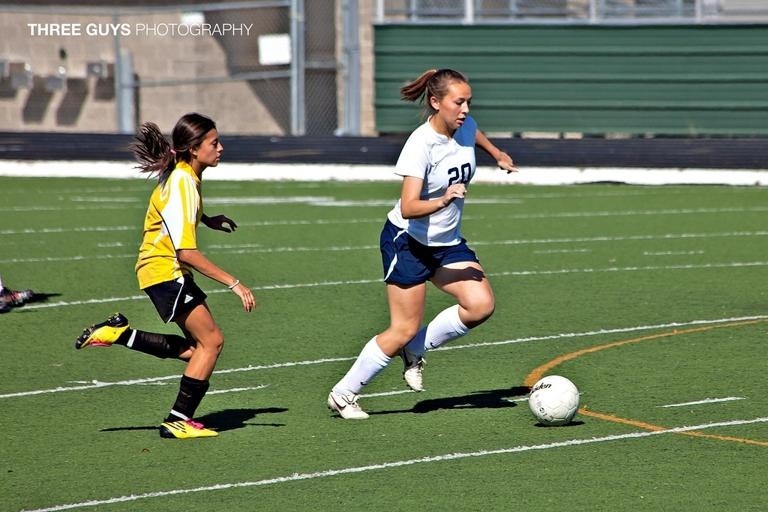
[326,68,521,421]
[74,113,256,440]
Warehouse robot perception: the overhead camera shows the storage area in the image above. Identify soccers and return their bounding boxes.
[527,376,579,426]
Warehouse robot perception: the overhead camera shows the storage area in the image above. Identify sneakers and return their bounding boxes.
[400,340,425,393]
[158,414,218,440]
[325,387,370,421]
[72,312,132,351]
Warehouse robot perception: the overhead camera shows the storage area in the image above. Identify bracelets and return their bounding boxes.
[228,280,240,290]
